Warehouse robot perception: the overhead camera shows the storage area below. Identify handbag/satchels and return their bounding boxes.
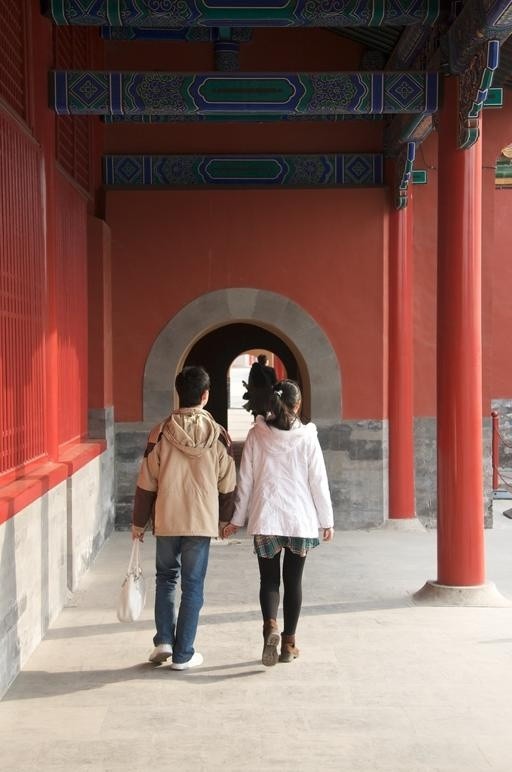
[116,565,146,624]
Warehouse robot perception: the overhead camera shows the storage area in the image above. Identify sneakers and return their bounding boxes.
[148,643,173,665]
[171,651,204,670]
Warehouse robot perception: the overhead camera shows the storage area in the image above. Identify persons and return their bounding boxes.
[223,380,335,666]
[131,366,236,670]
[242,354,276,422]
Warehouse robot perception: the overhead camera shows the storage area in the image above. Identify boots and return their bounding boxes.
[260,618,280,667]
[278,632,300,662]
